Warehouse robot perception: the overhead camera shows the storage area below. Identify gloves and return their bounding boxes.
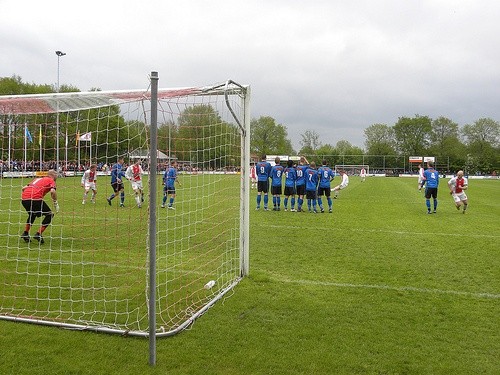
[116,177,121,183]
[53,200,59,213]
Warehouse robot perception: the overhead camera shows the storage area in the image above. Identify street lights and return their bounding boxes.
[56,51,67,175]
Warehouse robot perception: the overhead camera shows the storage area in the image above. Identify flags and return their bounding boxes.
[8,121,95,147]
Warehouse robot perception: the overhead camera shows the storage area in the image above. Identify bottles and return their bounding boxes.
[204,280,216,289]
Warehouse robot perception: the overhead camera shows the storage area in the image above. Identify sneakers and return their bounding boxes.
[34,232,44,243]
[82,200,85,204]
[21,231,32,242]
[119,204,125,207]
[161,204,175,210]
[107,198,111,206]
[457,207,460,210]
[255,207,332,213]
[138,203,143,208]
[334,195,337,199]
[90,200,95,203]
[427,210,436,214]
[463,210,467,214]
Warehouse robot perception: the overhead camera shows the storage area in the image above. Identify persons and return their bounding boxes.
[250,165,258,190]
[125,159,145,207]
[254,154,272,211]
[269,157,284,211]
[360,167,367,183]
[160,161,182,209]
[329,171,349,200]
[1,152,251,176]
[315,160,334,213]
[304,161,317,213]
[20,170,60,244]
[296,156,310,212]
[106,157,125,208]
[80,164,97,205]
[448,170,469,215]
[418,161,440,214]
[283,160,298,211]
[417,164,426,192]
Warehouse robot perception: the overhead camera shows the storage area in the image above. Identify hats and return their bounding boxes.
[458,170,463,176]
